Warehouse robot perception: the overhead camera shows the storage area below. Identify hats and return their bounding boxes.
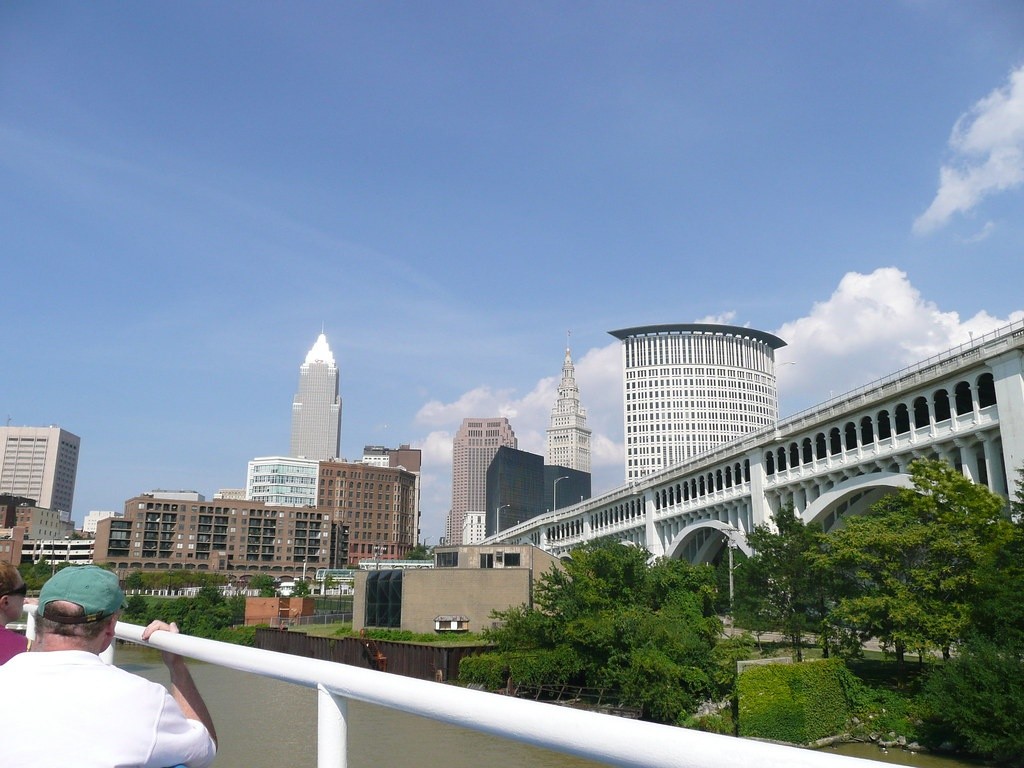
[37,565,129,624]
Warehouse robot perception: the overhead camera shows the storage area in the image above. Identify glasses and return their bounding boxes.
[0,583,27,599]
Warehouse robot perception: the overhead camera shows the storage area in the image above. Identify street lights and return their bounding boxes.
[497,505,510,534]
[770,362,797,431]
[553,475,569,520]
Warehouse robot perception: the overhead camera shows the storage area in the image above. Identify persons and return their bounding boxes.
[0,559,28,666]
[0,565,218,768]
